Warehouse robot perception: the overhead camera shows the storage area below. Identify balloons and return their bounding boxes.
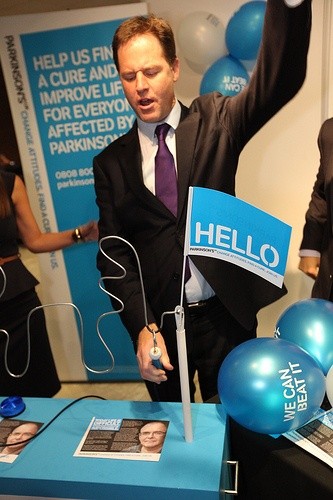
[217,336,326,434]
[199,55,249,97]
[326,365,333,408]
[225,0,267,60]
[274,298,333,377]
[176,11,225,75]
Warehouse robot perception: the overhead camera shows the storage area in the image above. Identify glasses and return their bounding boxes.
[12,433,33,437]
[139,431,161,436]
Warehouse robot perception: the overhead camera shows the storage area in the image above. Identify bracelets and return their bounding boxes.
[72,227,85,243]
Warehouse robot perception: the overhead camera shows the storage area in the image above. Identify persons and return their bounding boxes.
[120,422,166,453]
[93,0,312,404]
[0,423,38,455]
[0,152,99,398]
[298,117,333,303]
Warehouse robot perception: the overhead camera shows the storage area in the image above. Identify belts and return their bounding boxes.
[0,255,19,265]
[187,302,207,307]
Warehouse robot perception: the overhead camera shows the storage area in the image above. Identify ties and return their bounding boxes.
[154,123,191,285]
